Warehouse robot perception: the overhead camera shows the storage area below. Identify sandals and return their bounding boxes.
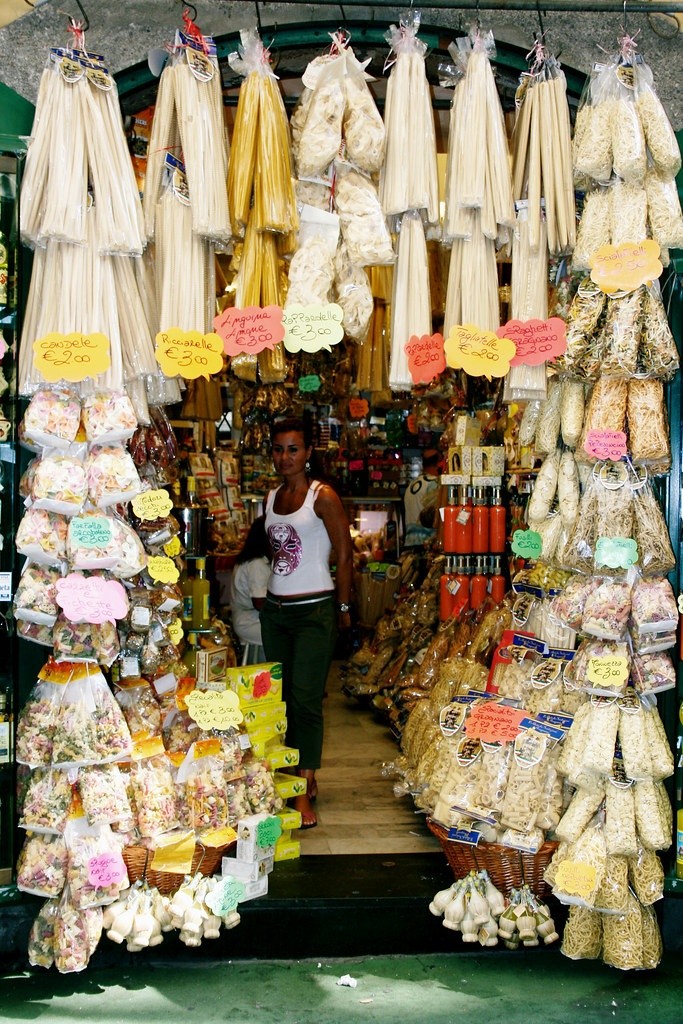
[298,797,317,830]
[301,779,320,801]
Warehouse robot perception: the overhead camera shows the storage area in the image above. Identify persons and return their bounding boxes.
[230,516,273,646]
[404,449,443,590]
[261,417,353,829]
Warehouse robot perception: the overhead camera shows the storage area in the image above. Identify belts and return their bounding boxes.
[262,596,332,607]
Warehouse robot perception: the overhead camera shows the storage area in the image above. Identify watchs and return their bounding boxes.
[340,603,352,612]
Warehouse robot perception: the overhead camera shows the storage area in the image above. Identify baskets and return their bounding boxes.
[425,813,561,901]
[121,840,233,895]
